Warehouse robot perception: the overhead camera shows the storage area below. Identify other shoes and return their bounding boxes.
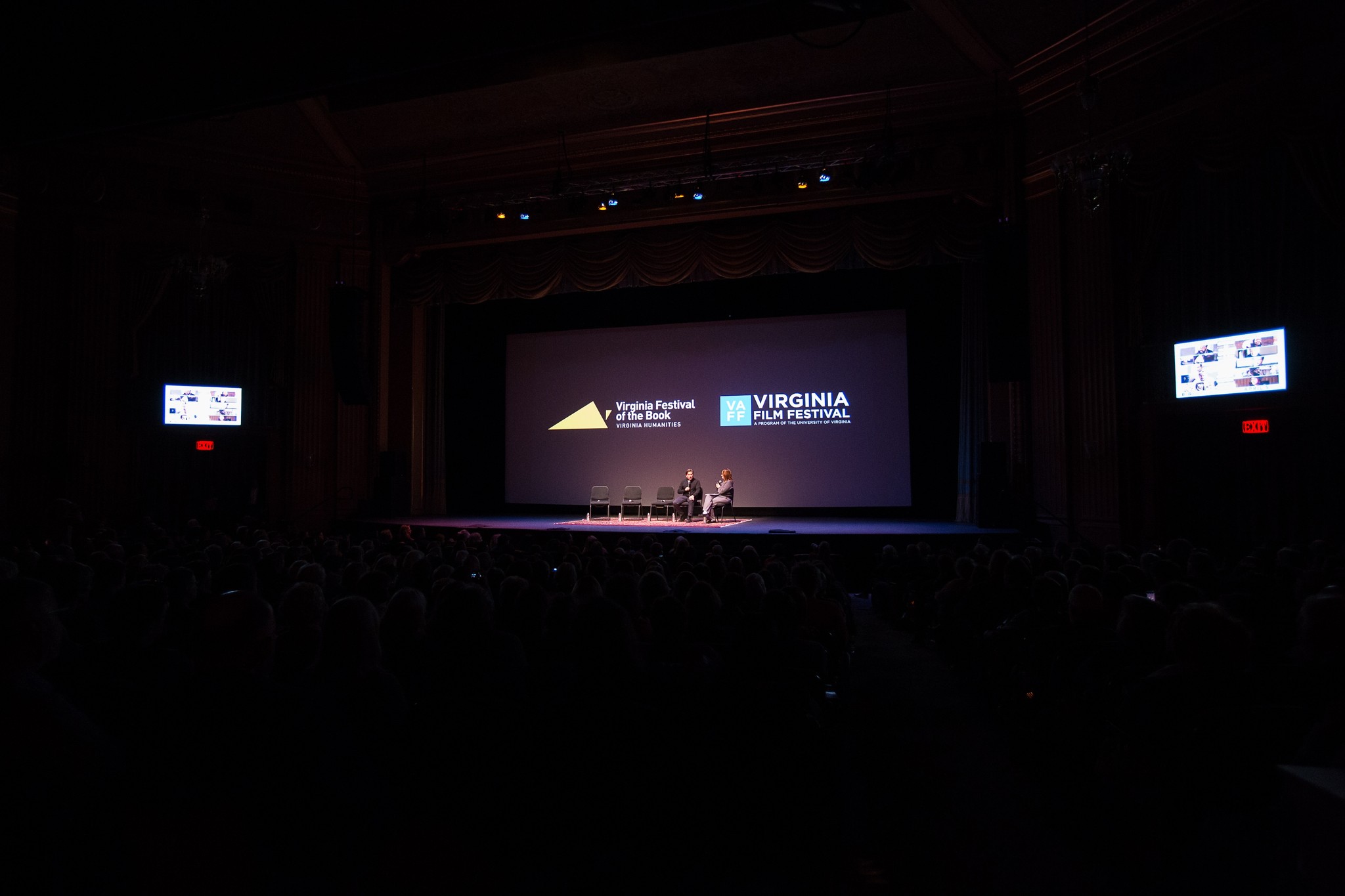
[706,519,717,523]
[679,513,688,522]
[686,518,692,522]
[698,513,709,517]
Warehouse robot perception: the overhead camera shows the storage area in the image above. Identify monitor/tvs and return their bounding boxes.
[1173,327,1287,398]
[164,384,242,427]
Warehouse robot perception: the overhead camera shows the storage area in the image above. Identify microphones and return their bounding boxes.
[688,480,690,487]
[716,480,722,490]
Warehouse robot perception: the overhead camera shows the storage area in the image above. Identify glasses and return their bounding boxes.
[721,473,723,475]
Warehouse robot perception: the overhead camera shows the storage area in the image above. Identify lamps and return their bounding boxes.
[608,192,618,206]
[597,199,607,211]
[817,166,830,182]
[520,203,529,220]
[675,185,685,199]
[691,183,706,201]
[796,170,808,189]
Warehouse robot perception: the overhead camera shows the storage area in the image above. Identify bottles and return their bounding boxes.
[672,513,675,522]
[586,513,590,521]
[618,513,621,522]
[703,517,707,523]
[647,513,650,522]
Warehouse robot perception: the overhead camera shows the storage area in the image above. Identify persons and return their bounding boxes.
[698,469,735,524]
[1,524,1344,896]
[169,390,232,422]
[673,469,698,523]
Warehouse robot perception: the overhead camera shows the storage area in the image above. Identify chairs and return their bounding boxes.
[714,489,736,523]
[676,486,703,522]
[650,486,676,522]
[621,486,643,521]
[590,486,610,521]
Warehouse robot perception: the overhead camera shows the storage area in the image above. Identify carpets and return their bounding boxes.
[553,517,752,527]
